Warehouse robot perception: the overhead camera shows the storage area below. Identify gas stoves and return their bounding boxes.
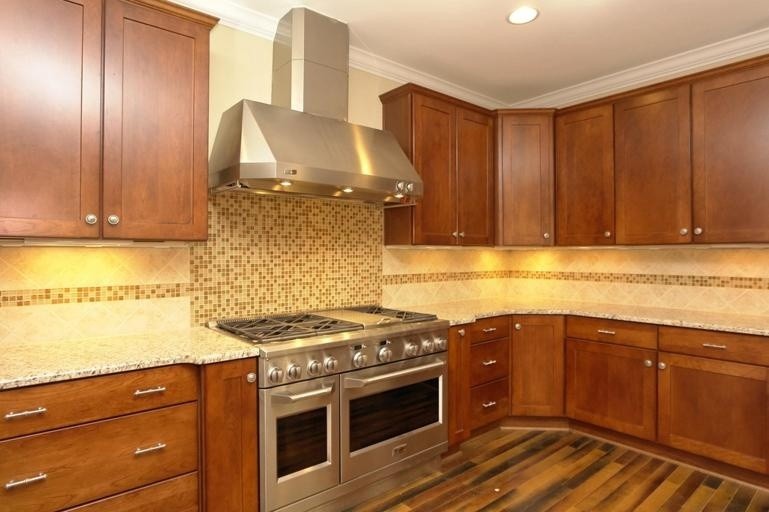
[207,304,451,353]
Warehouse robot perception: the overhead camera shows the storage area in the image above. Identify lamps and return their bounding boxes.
[506,5,542,24]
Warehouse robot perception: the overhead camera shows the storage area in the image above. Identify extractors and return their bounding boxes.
[207,8,424,209]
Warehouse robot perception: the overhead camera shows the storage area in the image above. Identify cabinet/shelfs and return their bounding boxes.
[445,325,471,448]
[0,362,200,512]
[0,0,220,238]
[381,84,498,247]
[493,109,556,245]
[457,315,514,437]
[614,54,769,245]
[512,315,564,418]
[557,96,617,245]
[180,357,259,511]
[567,316,769,490]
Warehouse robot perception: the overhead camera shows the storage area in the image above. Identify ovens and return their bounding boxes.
[259,329,449,511]
